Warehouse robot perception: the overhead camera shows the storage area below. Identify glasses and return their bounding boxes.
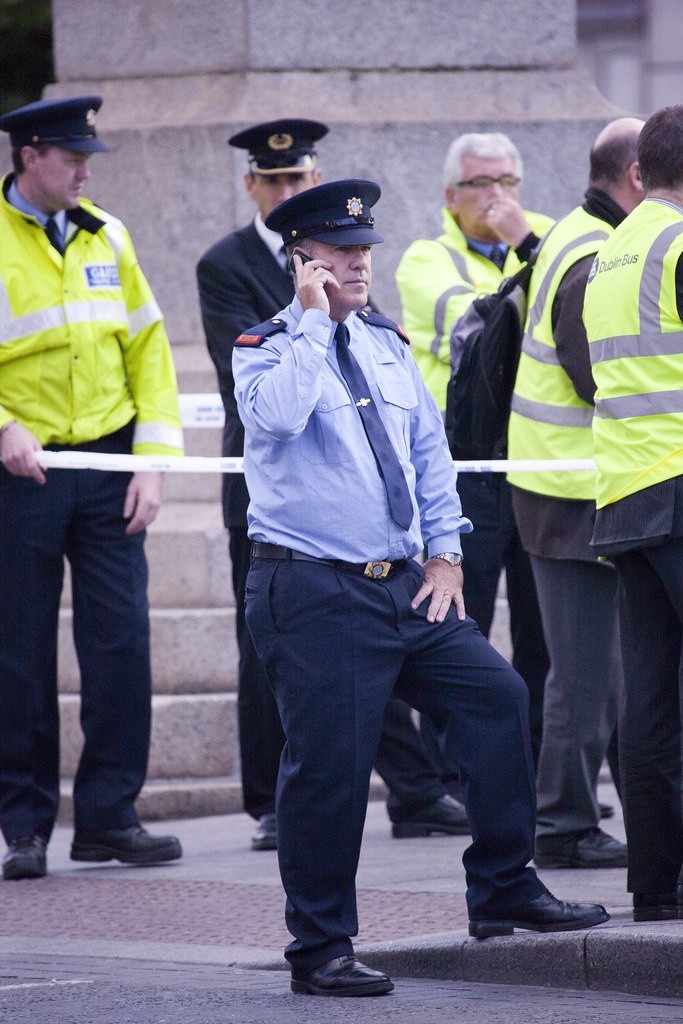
[457,171,521,189]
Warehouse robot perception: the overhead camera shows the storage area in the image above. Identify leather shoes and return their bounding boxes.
[392,795,468,837]
[533,828,627,868]
[468,890,612,937]
[2,835,48,879]
[633,888,683,922]
[291,954,394,996]
[71,824,182,862]
[252,814,275,849]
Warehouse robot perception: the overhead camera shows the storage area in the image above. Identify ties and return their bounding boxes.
[491,247,505,271]
[45,220,63,255]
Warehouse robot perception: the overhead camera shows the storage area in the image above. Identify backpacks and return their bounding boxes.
[444,222,559,486]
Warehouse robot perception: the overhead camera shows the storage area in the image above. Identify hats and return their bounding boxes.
[265,179,383,245]
[1,95,112,152]
[228,118,329,173]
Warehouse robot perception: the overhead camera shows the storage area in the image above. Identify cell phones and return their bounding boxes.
[289,251,317,274]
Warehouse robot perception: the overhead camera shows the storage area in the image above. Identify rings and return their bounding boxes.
[489,208,497,218]
[318,279,326,285]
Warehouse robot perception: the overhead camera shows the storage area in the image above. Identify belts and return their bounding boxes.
[252,543,407,578]
[335,323,414,533]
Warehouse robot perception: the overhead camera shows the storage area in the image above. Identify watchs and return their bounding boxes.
[434,552,462,567]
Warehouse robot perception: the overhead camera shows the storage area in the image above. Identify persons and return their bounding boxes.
[0,0,58,132]
[194,120,472,852]
[0,96,185,881]
[396,129,556,777]
[231,179,613,997]
[583,104,683,923]
[506,117,647,869]
